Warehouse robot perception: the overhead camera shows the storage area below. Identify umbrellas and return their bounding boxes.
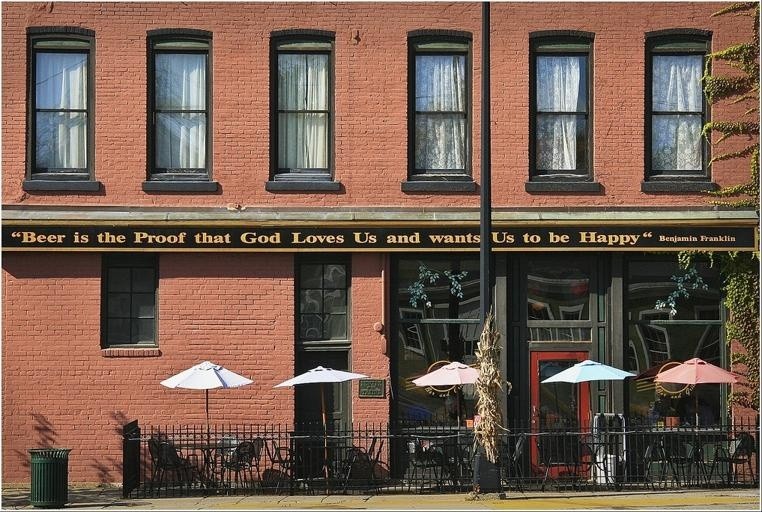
[539,357,639,444]
[410,361,481,444]
[653,356,739,436]
[271,364,371,495]
[159,360,254,482]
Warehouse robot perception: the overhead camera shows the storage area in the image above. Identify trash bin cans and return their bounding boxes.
[27,448,72,508]
